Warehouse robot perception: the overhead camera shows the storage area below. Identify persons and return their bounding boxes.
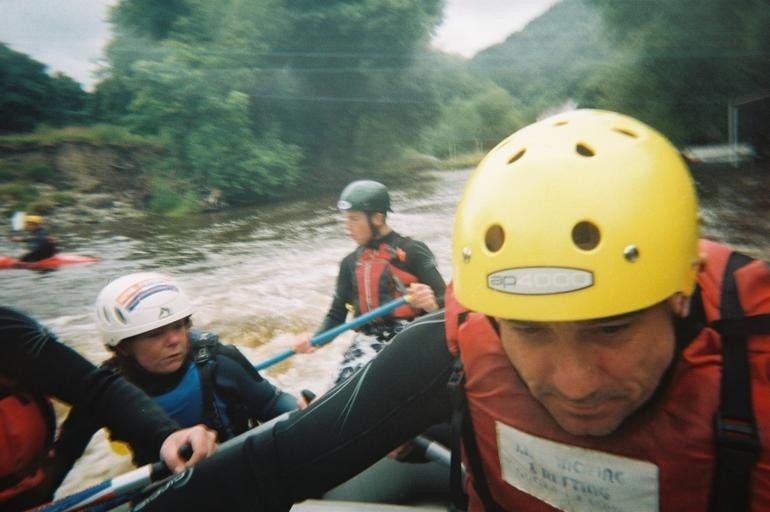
[114,106,770,511]
[45,268,467,510]
[7,212,63,263]
[290,174,451,391]
[0,302,221,510]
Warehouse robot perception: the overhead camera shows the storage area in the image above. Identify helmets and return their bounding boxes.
[24,214,44,225]
[336,180,392,214]
[91,270,194,348]
[448,105,702,326]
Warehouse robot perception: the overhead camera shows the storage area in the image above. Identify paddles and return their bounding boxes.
[8,207,21,240]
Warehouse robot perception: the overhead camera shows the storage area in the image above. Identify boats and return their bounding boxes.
[0,252,96,270]
